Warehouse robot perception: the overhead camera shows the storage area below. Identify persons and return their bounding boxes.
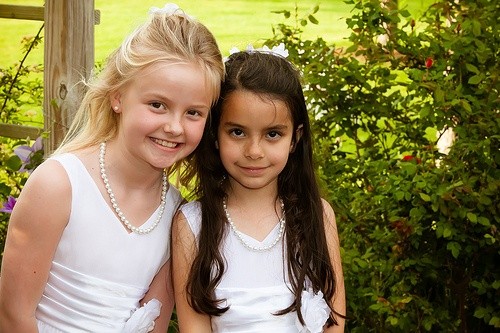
[0,4,222,333]
[172,52,346,333]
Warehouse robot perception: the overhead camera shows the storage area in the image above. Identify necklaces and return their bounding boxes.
[223,193,287,253]
[99,141,169,234]
